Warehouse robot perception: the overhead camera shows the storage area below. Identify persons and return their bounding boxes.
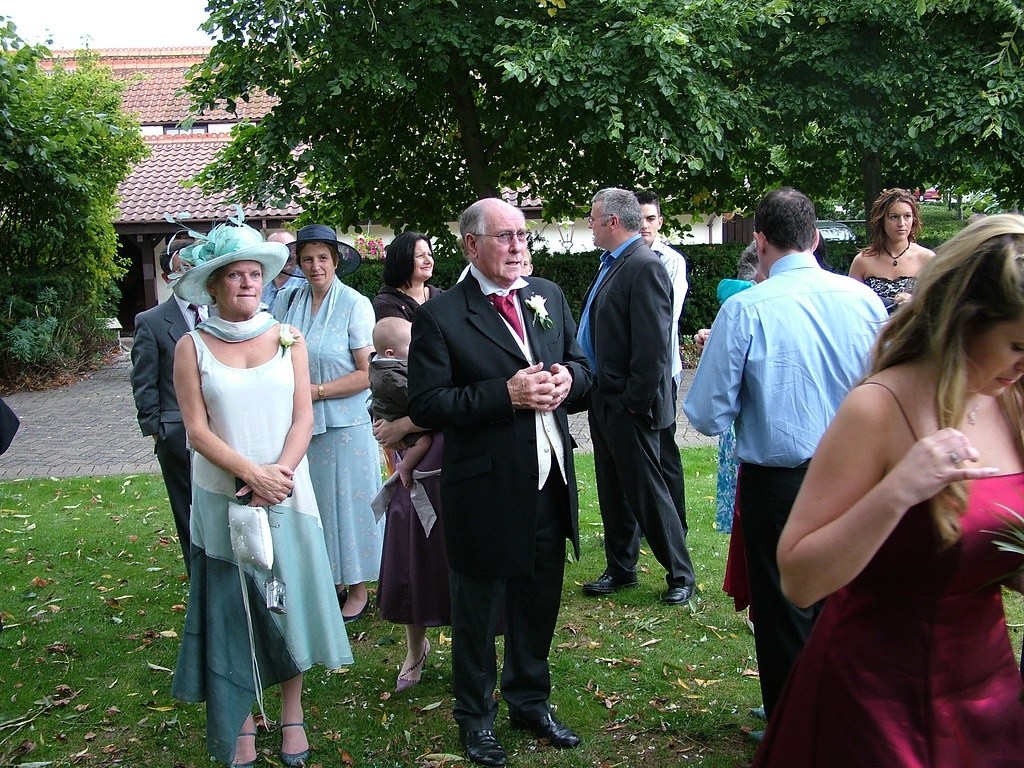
[260,232,309,318]
[682,188,892,746]
[407,198,594,768]
[694,233,846,636]
[365,263,471,694]
[130,240,220,580]
[749,214,1024,768]
[164,208,354,768]
[575,188,697,606]
[273,224,386,624]
[520,249,533,277]
[600,191,688,547]
[0,398,20,456]
[372,231,447,323]
[966,209,1020,227]
[848,188,936,316]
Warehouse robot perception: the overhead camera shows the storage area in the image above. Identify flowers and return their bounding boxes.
[277,330,301,358]
[525,294,555,331]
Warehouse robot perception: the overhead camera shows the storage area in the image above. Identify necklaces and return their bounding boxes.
[883,241,910,266]
[966,396,983,425]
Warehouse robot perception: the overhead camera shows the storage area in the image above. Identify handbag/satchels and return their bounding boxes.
[228,502,274,570]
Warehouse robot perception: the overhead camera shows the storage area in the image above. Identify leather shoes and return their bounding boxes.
[665,581,697,604]
[582,572,639,594]
[511,710,581,748]
[459,727,509,765]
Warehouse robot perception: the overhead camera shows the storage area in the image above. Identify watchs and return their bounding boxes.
[318,384,326,399]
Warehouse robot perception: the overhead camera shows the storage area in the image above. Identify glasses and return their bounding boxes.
[474,229,527,243]
[587,213,614,225]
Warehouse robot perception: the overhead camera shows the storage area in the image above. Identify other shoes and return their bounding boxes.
[281,720,310,768]
[337,588,348,607]
[342,592,369,623]
[231,726,258,768]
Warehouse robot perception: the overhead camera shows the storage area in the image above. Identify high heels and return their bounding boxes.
[396,637,431,691]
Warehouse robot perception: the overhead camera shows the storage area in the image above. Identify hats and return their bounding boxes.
[172,225,291,305]
[280,224,361,280]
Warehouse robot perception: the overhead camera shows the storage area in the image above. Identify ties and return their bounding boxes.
[188,303,202,329]
[488,291,525,345]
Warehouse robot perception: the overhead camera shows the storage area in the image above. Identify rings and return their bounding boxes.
[950,453,961,464]
